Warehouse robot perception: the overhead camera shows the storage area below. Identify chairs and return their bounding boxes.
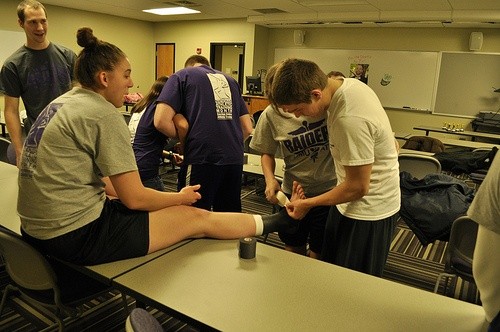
[0,228,131,332]
[432,215,484,297]
[392,153,441,215]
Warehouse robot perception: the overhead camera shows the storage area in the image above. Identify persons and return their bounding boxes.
[351,65,367,84]
[14,26,307,266]
[128,56,403,278]
[1,1,78,170]
[465,148,500,332]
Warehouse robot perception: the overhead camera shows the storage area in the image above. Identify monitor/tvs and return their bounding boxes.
[246,76,261,95]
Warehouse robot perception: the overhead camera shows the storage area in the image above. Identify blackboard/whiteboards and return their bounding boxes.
[274,48,439,114]
[432,50,500,120]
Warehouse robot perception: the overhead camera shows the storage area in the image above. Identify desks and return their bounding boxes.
[238,153,286,242]
[0,162,493,332]
[238,93,274,130]
[394,126,500,182]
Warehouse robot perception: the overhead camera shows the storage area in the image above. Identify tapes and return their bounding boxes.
[275,190,291,207]
[239,236,257,260]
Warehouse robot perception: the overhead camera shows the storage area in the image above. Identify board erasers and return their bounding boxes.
[402,106,410,108]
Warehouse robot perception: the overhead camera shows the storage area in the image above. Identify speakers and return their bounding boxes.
[469,32,483,50]
[294,30,304,45]
